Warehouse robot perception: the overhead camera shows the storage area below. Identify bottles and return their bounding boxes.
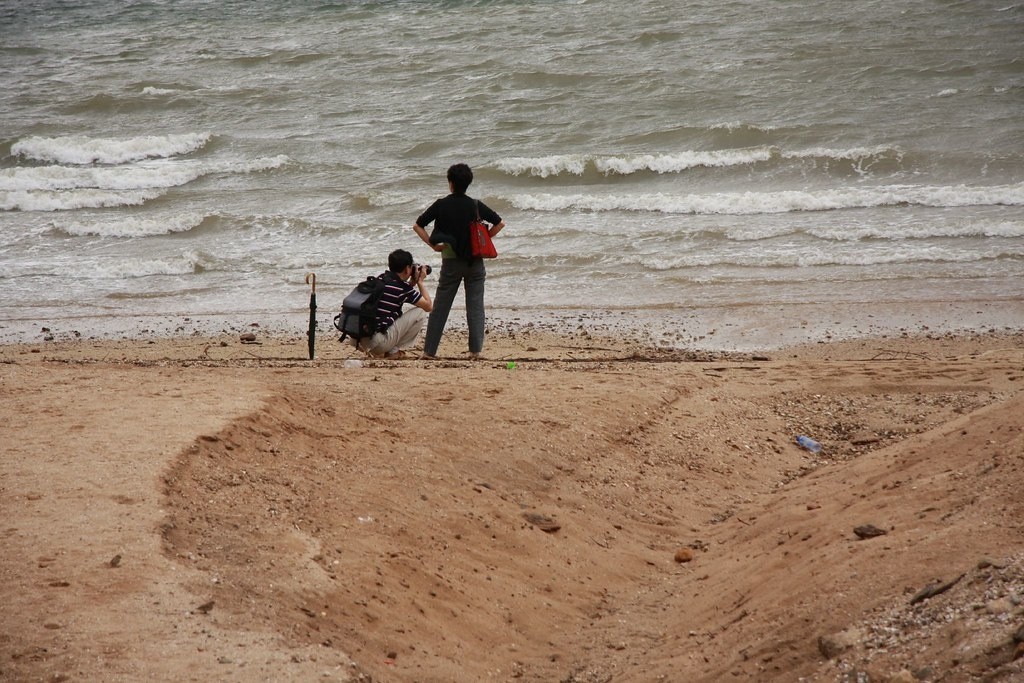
[345,360,363,368]
[796,435,822,452]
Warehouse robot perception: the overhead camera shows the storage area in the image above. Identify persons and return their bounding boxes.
[412,163,506,359]
[350,249,433,361]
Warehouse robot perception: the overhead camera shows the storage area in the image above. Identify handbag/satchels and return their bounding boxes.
[469,199,498,258]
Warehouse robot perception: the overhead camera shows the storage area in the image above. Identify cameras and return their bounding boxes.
[410,263,432,277]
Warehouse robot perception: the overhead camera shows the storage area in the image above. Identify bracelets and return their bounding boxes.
[427,242,431,245]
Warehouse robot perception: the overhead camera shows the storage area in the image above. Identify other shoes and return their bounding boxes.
[468,354,488,360]
[367,350,384,360]
[387,350,408,360]
[418,354,438,360]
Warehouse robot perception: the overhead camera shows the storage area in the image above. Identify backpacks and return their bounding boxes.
[334,274,397,350]
